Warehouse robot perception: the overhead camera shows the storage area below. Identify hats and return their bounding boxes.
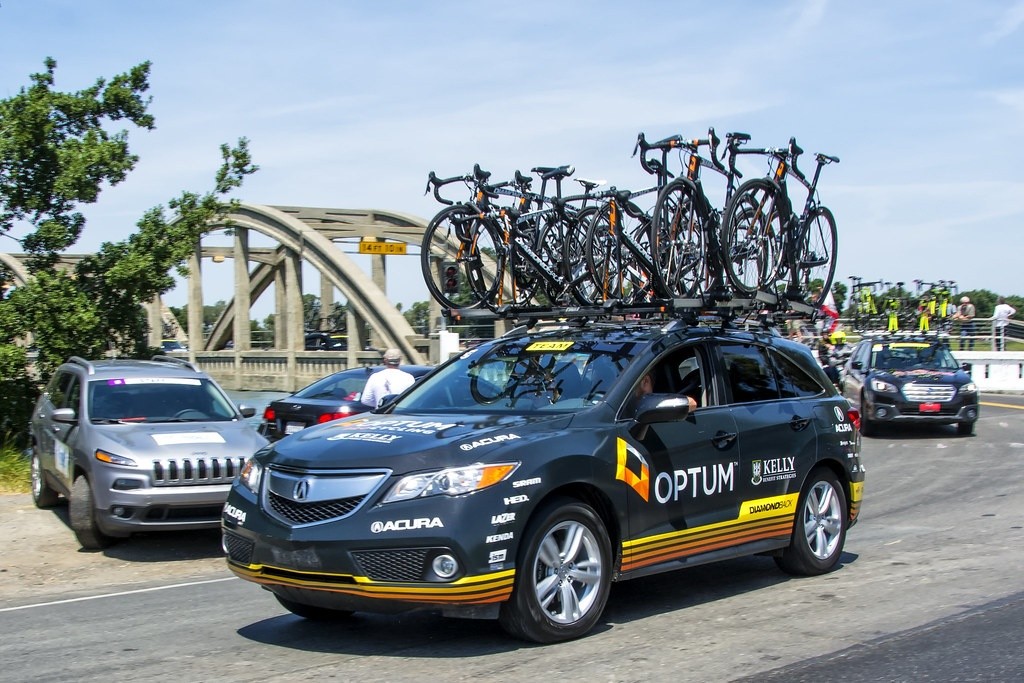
[383,349,402,364]
[960,296,970,303]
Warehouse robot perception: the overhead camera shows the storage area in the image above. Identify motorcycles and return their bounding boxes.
[817,352,853,377]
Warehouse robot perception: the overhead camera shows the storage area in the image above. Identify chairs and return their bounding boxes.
[653,360,692,401]
[546,362,583,405]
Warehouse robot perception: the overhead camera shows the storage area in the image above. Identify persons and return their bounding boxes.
[828,332,857,383]
[787,279,839,349]
[942,299,957,350]
[989,296,1016,351]
[633,369,697,412]
[948,296,976,351]
[362,350,415,410]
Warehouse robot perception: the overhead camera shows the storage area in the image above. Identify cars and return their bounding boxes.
[303,332,348,351]
[161,339,189,350]
[256,365,506,443]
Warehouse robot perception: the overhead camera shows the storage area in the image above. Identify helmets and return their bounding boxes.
[820,327,829,334]
[828,331,847,345]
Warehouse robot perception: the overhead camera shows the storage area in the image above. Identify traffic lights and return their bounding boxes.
[439,260,463,295]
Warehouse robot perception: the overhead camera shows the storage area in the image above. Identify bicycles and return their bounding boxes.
[847,275,958,333]
[419,165,656,311]
[630,128,839,307]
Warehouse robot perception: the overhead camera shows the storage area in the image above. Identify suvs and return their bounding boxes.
[838,333,981,437]
[27,355,276,551]
[220,310,869,644]
